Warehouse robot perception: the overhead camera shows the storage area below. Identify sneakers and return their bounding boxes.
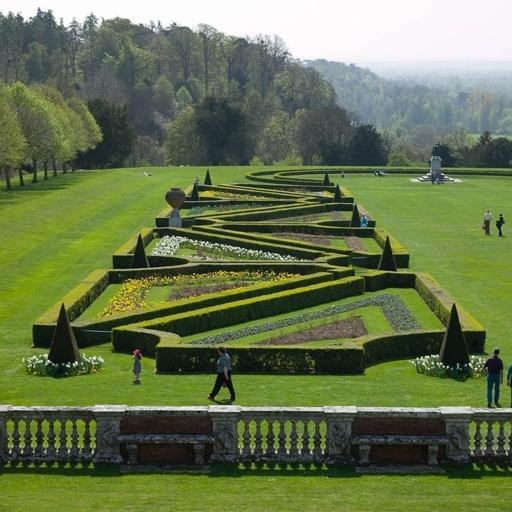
[206,394,235,401]
[487,401,499,407]
[485,232,502,237]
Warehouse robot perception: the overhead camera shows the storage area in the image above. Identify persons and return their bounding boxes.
[132,348,142,382]
[483,210,492,236]
[496,213,504,236]
[505,362,512,403]
[140,168,148,177]
[483,347,503,406]
[206,346,235,402]
[360,214,368,228]
[430,171,443,184]
[374,169,385,177]
[341,170,345,177]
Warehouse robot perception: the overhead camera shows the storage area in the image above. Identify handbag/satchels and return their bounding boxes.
[482,224,486,230]
